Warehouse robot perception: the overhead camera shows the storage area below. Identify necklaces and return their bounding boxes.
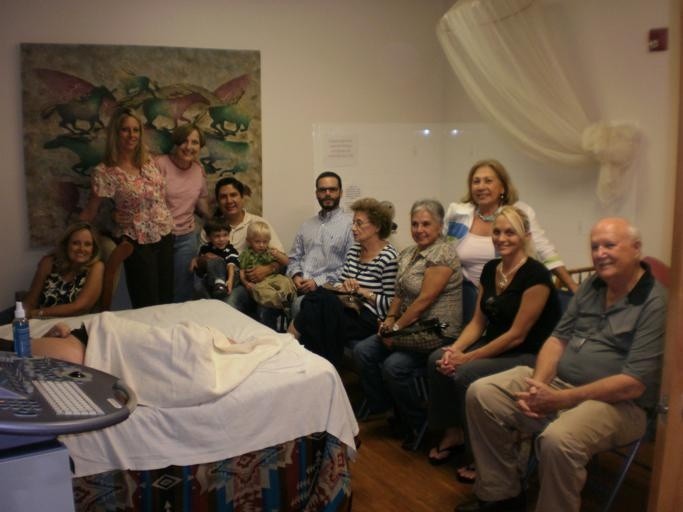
[476,206,500,222]
[495,256,526,290]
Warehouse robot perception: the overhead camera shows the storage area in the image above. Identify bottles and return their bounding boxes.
[11,300,33,359]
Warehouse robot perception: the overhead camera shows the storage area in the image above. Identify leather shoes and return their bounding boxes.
[402,427,444,450]
[455,491,527,512]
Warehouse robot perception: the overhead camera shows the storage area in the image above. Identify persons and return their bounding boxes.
[238,222,294,310]
[443,156,580,330]
[22,220,103,317]
[109,124,212,301]
[0,311,88,367]
[197,176,285,330]
[288,197,398,360]
[428,205,563,485]
[79,108,175,308]
[349,199,463,414]
[189,217,241,296]
[286,172,357,313]
[445,215,667,512]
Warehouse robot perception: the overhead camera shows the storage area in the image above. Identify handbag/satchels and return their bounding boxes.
[322,281,364,315]
[381,317,448,351]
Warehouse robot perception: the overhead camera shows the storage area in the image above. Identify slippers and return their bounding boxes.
[428,441,466,464]
[459,464,477,484]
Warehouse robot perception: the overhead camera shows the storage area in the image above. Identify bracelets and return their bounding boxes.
[37,307,44,316]
[385,315,396,318]
[370,291,374,300]
[392,323,399,331]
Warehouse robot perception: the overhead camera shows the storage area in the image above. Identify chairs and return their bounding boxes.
[95,236,134,312]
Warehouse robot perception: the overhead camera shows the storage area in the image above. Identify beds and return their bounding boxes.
[0,298,359,512]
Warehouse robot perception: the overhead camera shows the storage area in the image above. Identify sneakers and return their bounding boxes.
[213,283,228,295]
